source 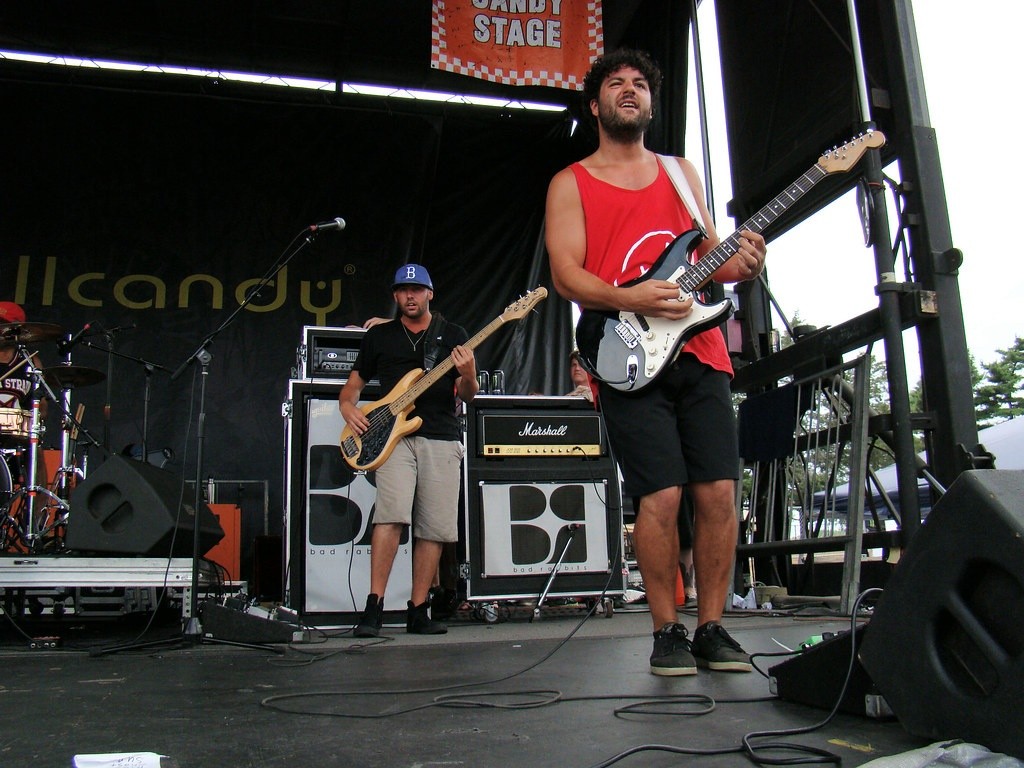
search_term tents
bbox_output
[812,415,1024,531]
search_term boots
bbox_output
[350,594,385,637]
[403,599,449,633]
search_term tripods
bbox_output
[90,232,321,661]
[0,335,87,557]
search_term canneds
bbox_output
[491,369,506,395]
[477,370,490,394]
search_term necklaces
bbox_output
[401,318,432,351]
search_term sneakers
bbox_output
[691,621,754,672]
[684,586,699,608]
[646,621,700,675]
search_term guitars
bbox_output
[572,125,891,395]
[339,282,550,473]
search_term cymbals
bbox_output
[40,364,109,389]
[0,322,65,349]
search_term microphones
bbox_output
[58,321,97,357]
[564,523,581,531]
[308,218,346,232]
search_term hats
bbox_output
[391,264,435,290]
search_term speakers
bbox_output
[280,376,432,628]
[450,395,628,601]
[64,452,225,560]
[856,469,1024,759]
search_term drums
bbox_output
[0,407,46,441]
[0,455,14,528]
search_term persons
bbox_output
[563,350,594,405]
[338,262,479,635]
[0,298,48,541]
[546,49,768,676]
